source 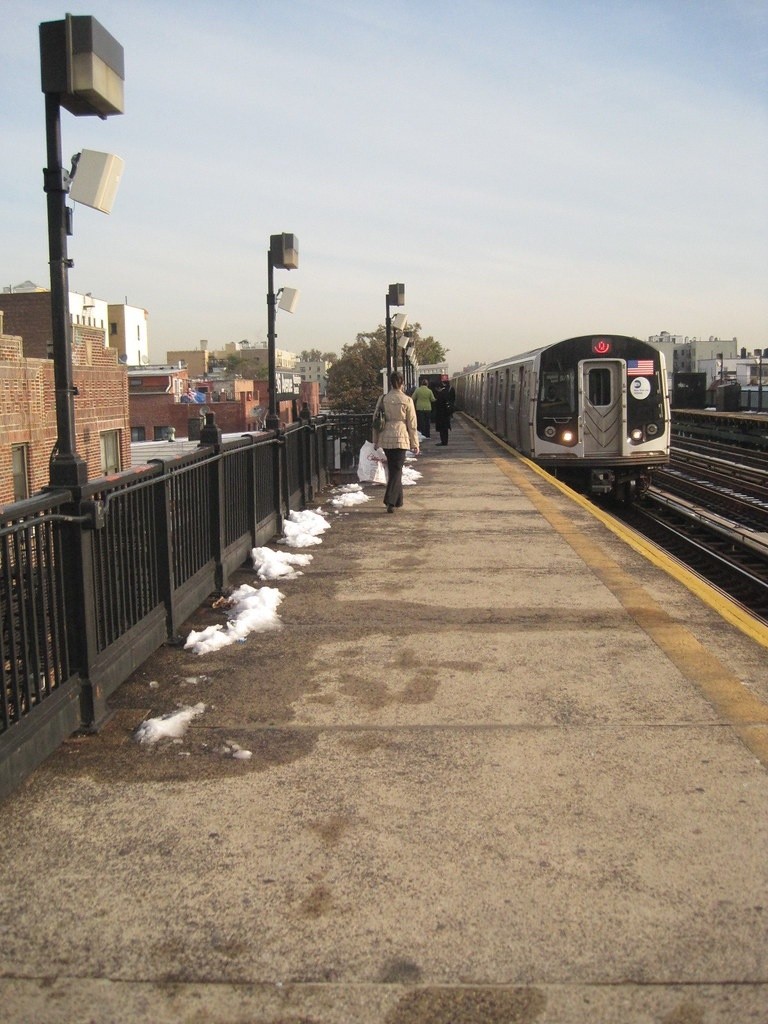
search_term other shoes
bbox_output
[435,442,445,446]
[387,502,393,513]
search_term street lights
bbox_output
[716,352,723,384]
[38,11,127,490]
[405,342,420,390]
[397,330,413,386]
[754,348,763,412]
[385,283,408,393]
[265,232,300,431]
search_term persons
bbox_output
[373,372,420,514]
[433,383,450,446]
[412,379,436,439]
[406,380,455,420]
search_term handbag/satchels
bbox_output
[358,440,388,487]
[372,394,386,433]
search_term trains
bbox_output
[449,334,673,504]
